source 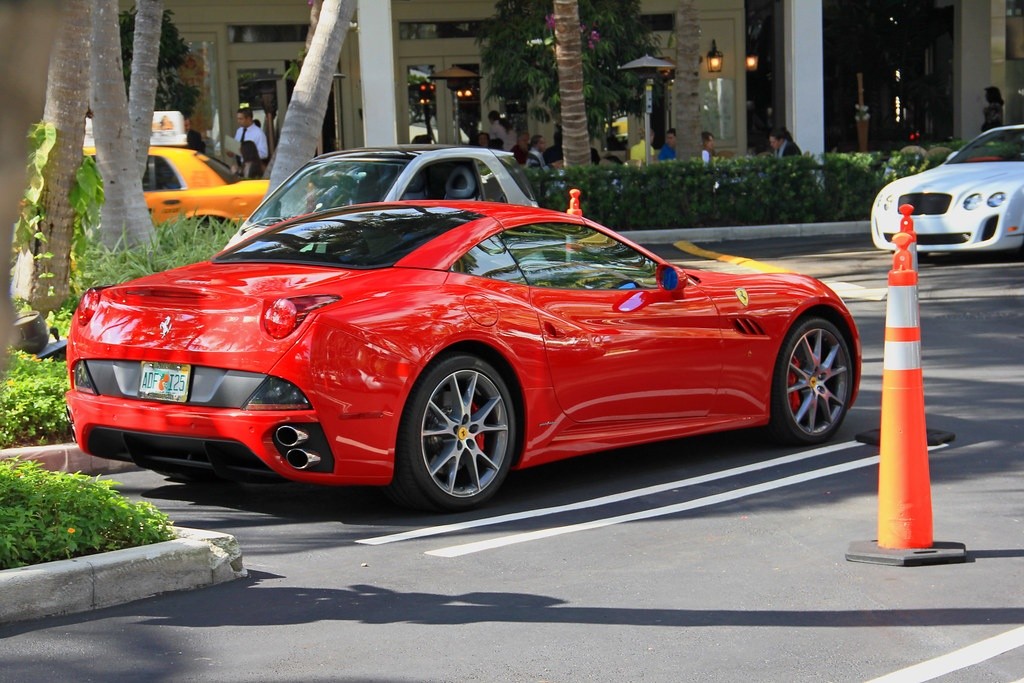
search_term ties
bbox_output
[240,127,247,143]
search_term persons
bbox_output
[183,117,207,155]
[701,132,716,165]
[224,107,269,179]
[629,127,655,164]
[981,86,1004,133]
[657,128,676,160]
[468,110,600,172]
[769,128,801,157]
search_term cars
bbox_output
[872,124,1024,266]
[84,111,269,234]
[222,145,540,253]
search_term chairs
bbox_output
[443,165,479,200]
[398,162,431,200]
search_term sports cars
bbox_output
[65,199,864,515]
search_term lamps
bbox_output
[427,64,483,91]
[707,39,723,73]
[745,48,759,69]
[241,73,285,93]
[619,53,676,79]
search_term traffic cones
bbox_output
[844,202,969,567]
[562,186,584,259]
